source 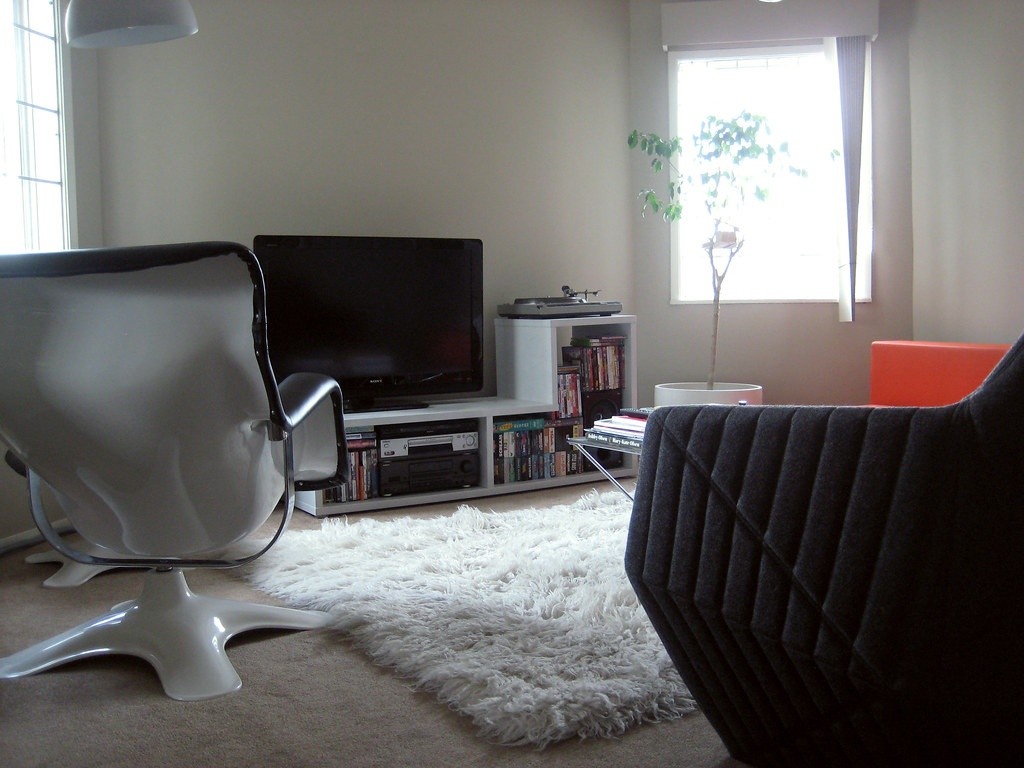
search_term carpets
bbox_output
[225,481,700,746]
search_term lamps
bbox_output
[64,0,199,47]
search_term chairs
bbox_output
[0,240,348,703]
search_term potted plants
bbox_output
[627,112,800,408]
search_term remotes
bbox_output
[621,407,652,418]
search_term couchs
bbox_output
[622,330,1024,768]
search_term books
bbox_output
[494,412,584,485]
[322,432,378,503]
[584,415,647,450]
[556,336,628,419]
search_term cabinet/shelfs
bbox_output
[294,313,638,519]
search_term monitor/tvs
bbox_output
[252,235,483,414]
